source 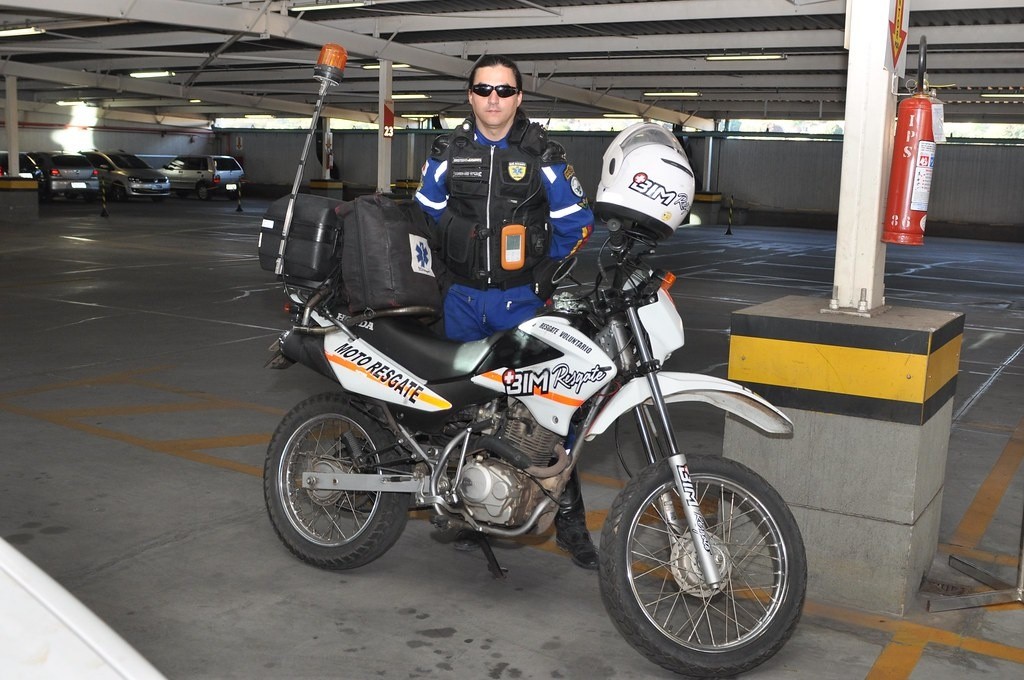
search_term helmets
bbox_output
[595,123,694,236]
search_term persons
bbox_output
[412,54,599,570]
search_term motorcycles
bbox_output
[255,43,810,680]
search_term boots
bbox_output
[545,457,602,570]
[454,520,489,551]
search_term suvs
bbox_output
[77,149,172,203]
[0,149,99,204]
[155,153,245,201]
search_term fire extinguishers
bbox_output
[881,36,945,246]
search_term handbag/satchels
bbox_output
[343,194,444,313]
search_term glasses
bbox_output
[471,83,518,98]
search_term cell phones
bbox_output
[501,225,525,270]
